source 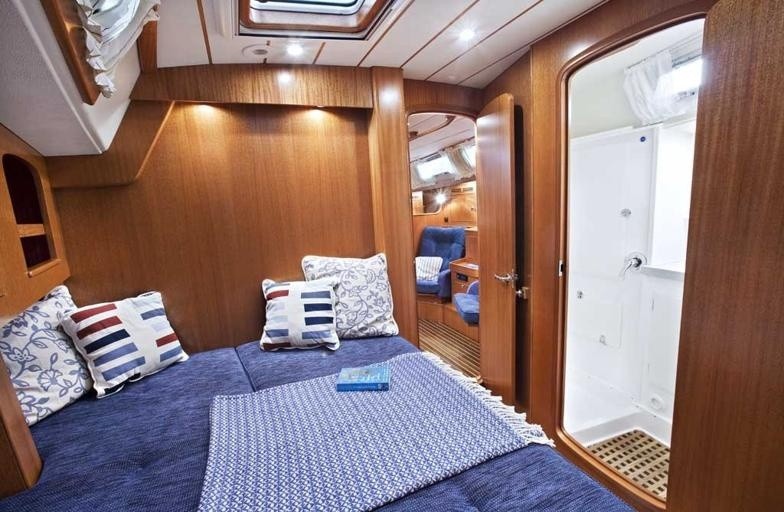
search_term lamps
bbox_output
[653,59,701,103]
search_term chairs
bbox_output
[454,281,483,324]
[416,226,465,299]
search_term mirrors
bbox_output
[642,120,699,279]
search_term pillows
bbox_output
[416,256,443,283]
[257,253,400,354]
[1,283,189,426]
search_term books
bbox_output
[336,365,390,391]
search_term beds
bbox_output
[0,318,637,510]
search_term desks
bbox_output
[449,257,481,306]
